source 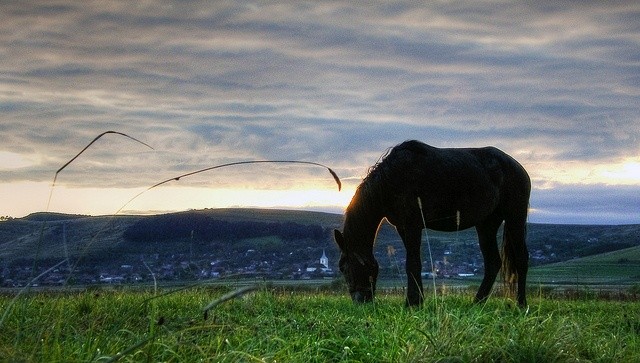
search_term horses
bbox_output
[334,139,531,309]
